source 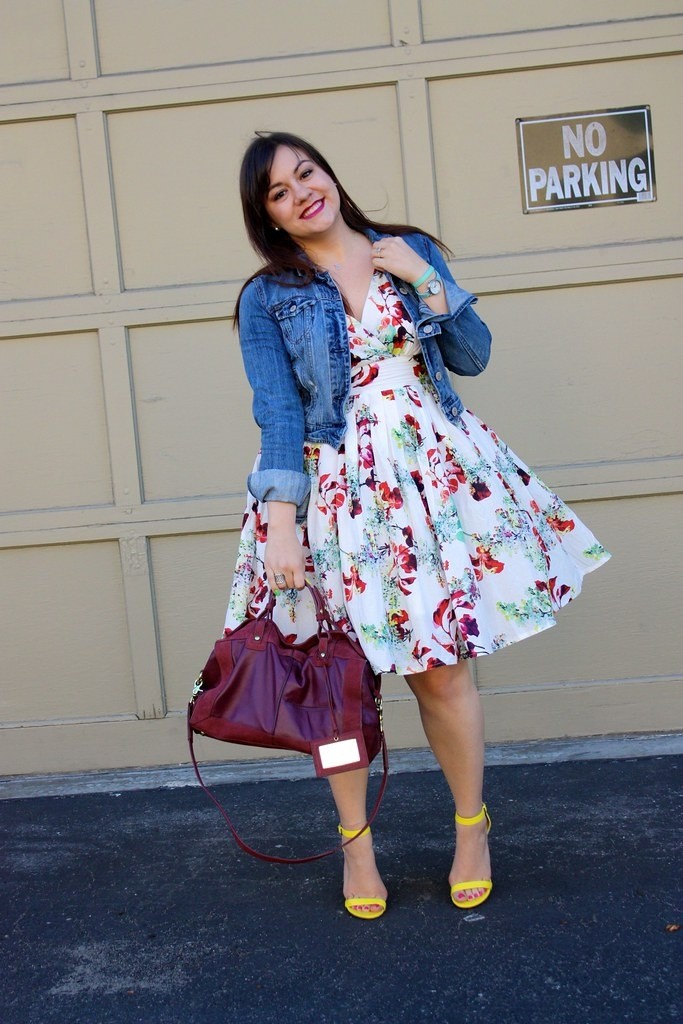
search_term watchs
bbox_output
[415,271,443,297]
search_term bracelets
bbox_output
[413,266,434,287]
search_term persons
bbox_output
[223,131,612,919]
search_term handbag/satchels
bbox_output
[186,579,388,865]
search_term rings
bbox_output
[275,574,283,583]
[375,247,382,257]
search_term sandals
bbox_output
[449,801,492,908]
[337,821,388,920]
